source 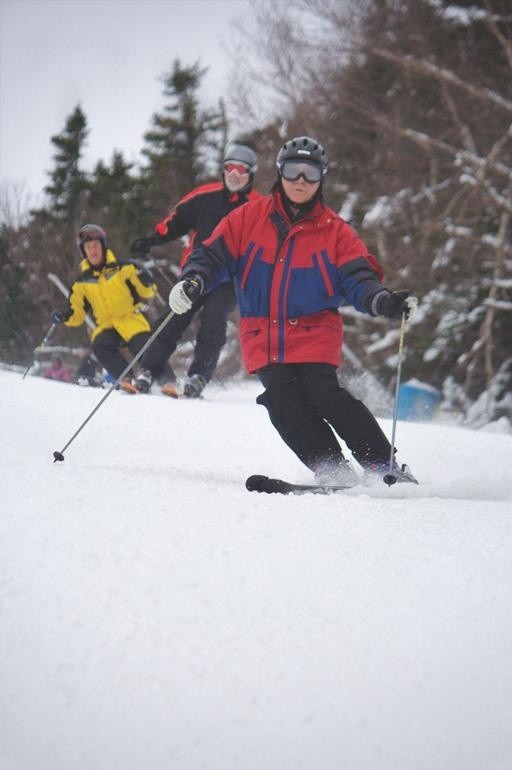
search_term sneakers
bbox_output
[113,368,210,397]
[315,455,361,485]
[362,457,402,485]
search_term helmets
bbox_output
[276,136,329,172]
[77,223,108,256]
[223,143,261,178]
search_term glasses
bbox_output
[276,158,326,183]
[225,159,258,174]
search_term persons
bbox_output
[130,143,266,397]
[73,348,98,387]
[51,223,185,398]
[44,356,69,383]
[169,136,423,488]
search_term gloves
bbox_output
[373,288,418,322]
[139,259,160,285]
[128,231,163,259]
[168,272,203,316]
[51,306,75,323]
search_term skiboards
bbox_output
[245,474,353,495]
[120,376,179,398]
